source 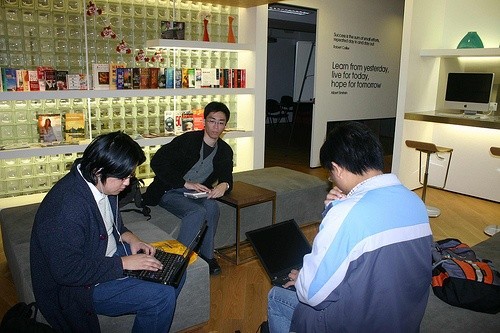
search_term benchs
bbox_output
[420,231,500,333]
[0,165,329,333]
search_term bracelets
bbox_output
[227,182,229,190]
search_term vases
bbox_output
[203,19,210,41]
[228,16,237,43]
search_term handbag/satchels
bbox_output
[431,238,500,314]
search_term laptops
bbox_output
[245,219,312,291]
[122,219,209,289]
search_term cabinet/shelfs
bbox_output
[0,38,257,206]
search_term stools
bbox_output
[482,146,500,236]
[214,180,277,266]
[406,139,454,216]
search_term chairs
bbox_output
[265,94,294,135]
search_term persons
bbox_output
[268,122,432,333]
[43,119,57,142]
[150,101,233,275]
[30,130,187,333]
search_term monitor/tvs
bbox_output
[444,72,495,112]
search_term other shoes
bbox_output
[199,254,220,272]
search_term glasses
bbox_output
[122,171,136,181]
[327,168,334,182]
[207,120,225,125]
